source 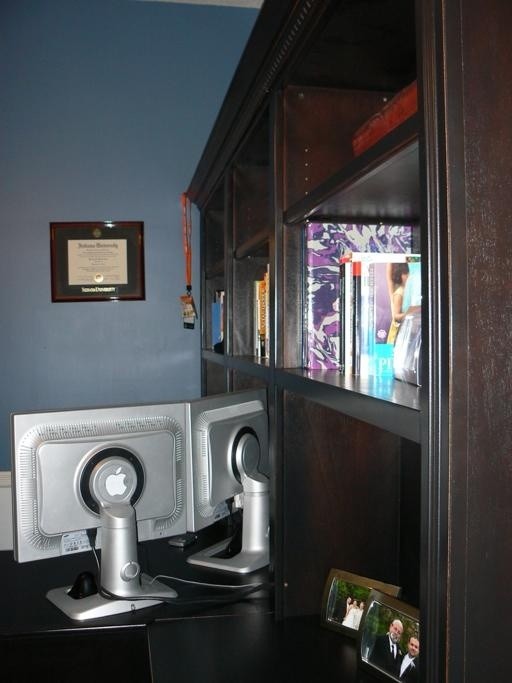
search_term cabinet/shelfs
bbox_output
[185,0,512,683]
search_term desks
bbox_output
[0,518,275,640]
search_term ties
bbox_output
[392,645,394,658]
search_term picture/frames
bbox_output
[319,568,402,641]
[49,221,145,303]
[357,589,420,683]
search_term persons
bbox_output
[367,618,406,679]
[390,635,418,683]
[340,599,360,628]
[331,595,353,623]
[351,601,367,630]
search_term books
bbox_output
[212,220,422,378]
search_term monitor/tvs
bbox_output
[185,385,271,575]
[9,399,188,621]
